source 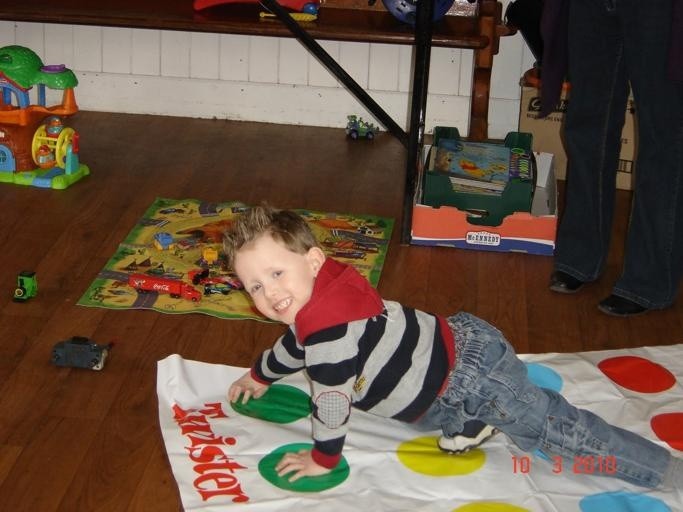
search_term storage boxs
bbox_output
[516,77,639,191]
[411,144,558,257]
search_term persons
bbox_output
[536,0,683,317]
[227,200,683,493]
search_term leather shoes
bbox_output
[597,295,648,317]
[550,271,584,294]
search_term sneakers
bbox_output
[437,420,501,455]
